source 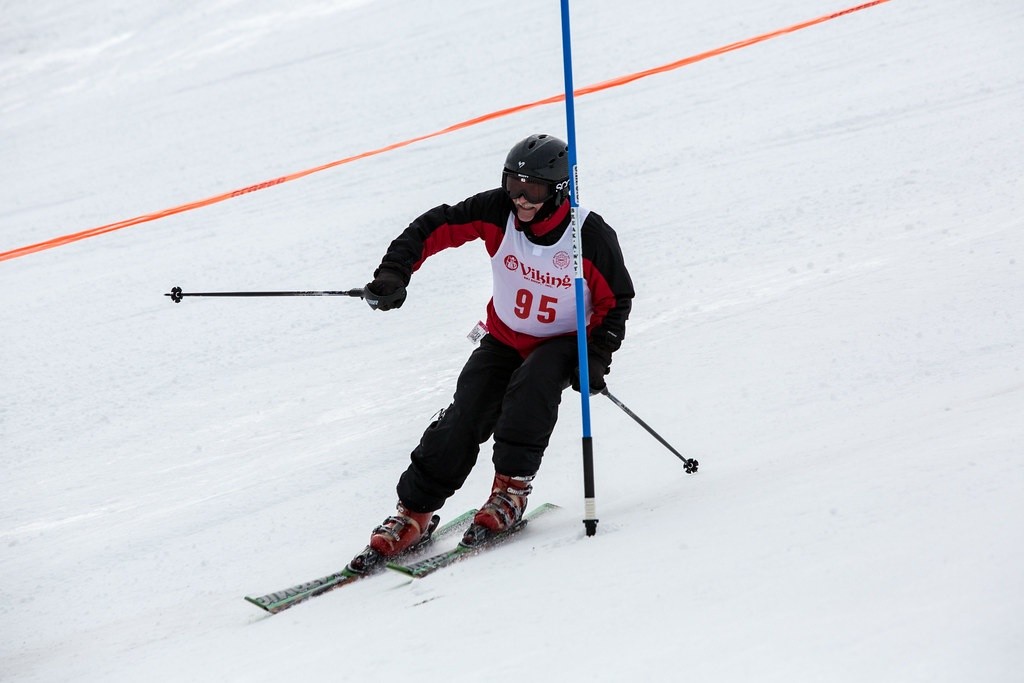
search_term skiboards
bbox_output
[244,502,567,617]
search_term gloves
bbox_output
[572,358,610,396]
[363,273,407,311]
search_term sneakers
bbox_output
[474,472,535,533]
[370,499,433,556]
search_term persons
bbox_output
[370,134,636,557]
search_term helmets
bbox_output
[504,134,570,195]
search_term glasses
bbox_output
[506,176,554,204]
